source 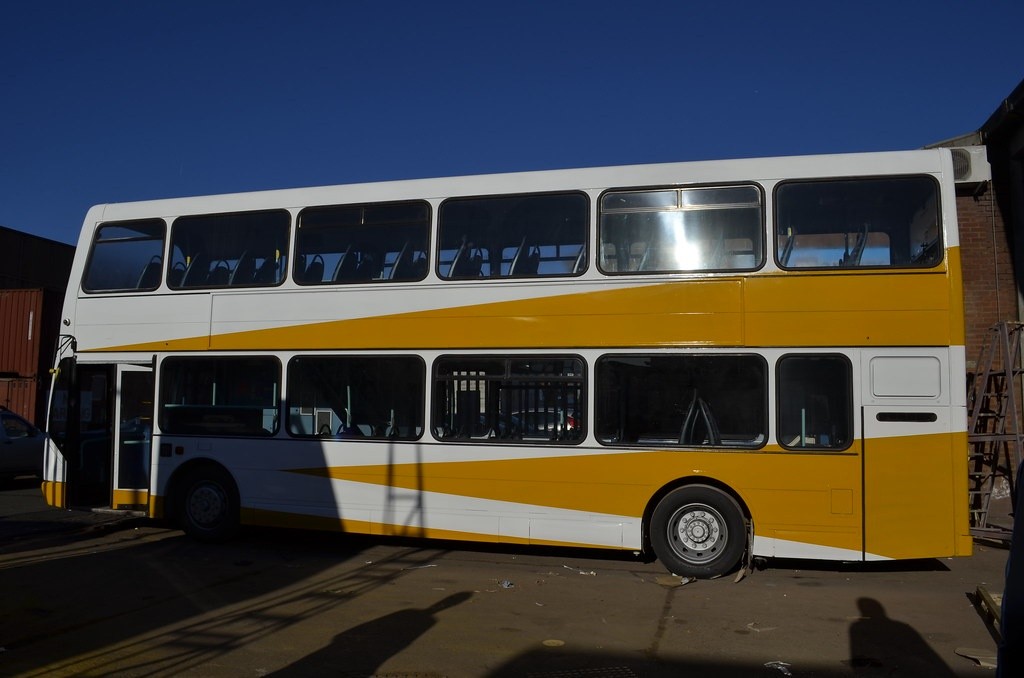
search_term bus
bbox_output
[41,150,972,581]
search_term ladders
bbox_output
[966,321,1024,542]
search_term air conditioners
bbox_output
[938,145,992,184]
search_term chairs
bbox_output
[129,215,871,443]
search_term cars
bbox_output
[1,405,43,478]
[511,410,581,432]
